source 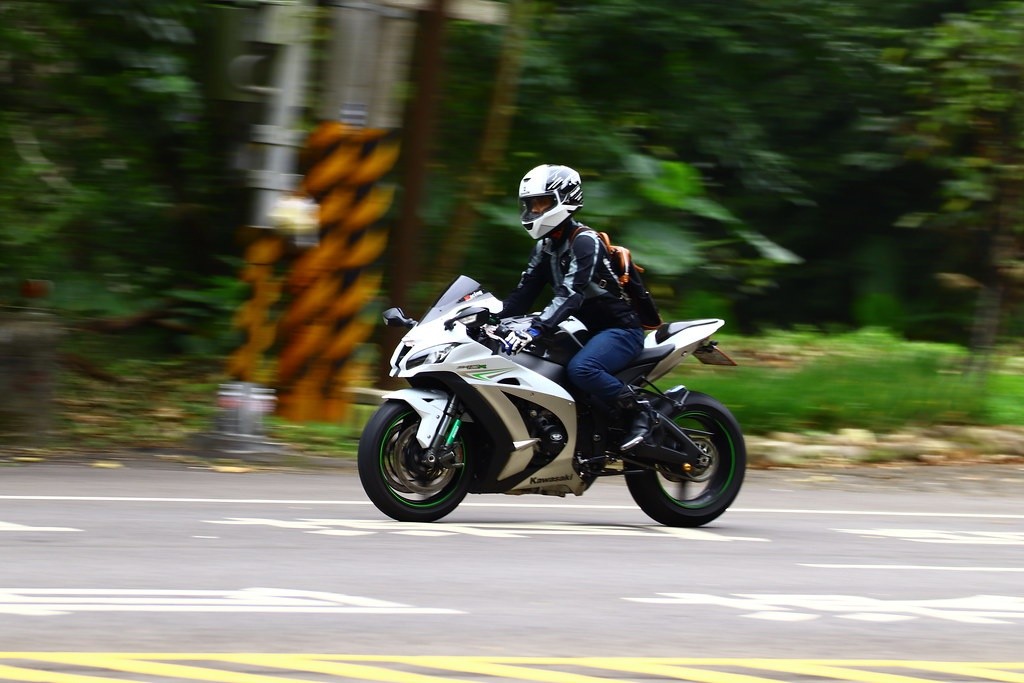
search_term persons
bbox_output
[499,164,660,453]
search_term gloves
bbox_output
[501,327,541,356]
[457,289,485,304]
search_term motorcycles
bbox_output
[357,275,746,528]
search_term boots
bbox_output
[610,384,662,451]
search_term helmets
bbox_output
[518,164,584,241]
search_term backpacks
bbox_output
[571,226,663,329]
